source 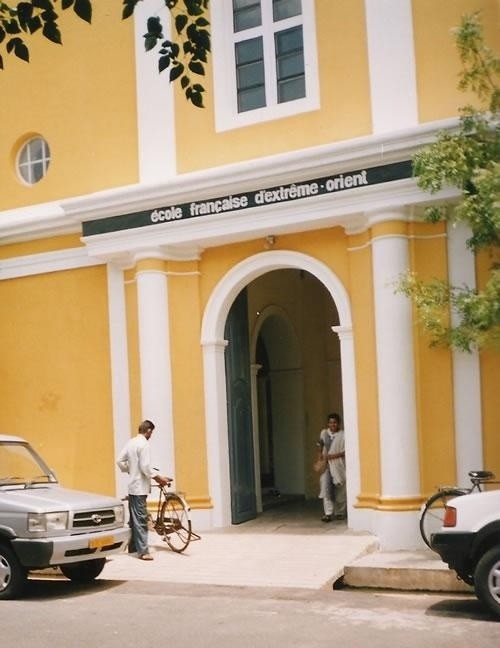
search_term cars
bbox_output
[429,489,500,618]
[0,435,126,599]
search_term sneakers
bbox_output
[321,515,345,521]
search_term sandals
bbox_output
[139,553,154,560]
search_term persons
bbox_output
[313,411,346,523]
[115,418,170,560]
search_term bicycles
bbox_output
[418,469,500,552]
[123,468,201,553]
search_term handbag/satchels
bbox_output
[313,458,327,473]
